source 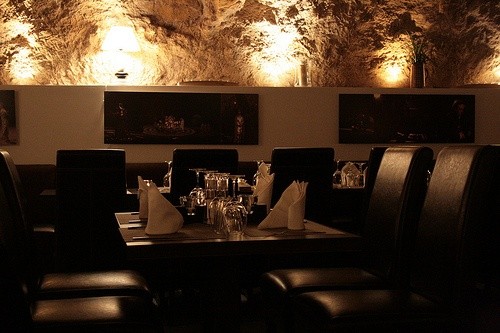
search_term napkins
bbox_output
[258,180,309,230]
[341,162,360,187]
[137,176,184,235]
[254,172,275,213]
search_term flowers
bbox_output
[387,12,445,64]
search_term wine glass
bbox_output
[189,168,248,237]
[164,160,171,189]
[355,162,366,188]
[333,158,342,187]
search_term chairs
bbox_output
[298,144,500,333]
[271,147,335,226]
[170,149,239,207]
[362,146,389,205]
[0,184,147,333]
[54,149,128,272]
[258,143,435,333]
[0,149,150,301]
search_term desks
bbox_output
[127,185,364,214]
[115,212,361,333]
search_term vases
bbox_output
[409,60,425,89]
